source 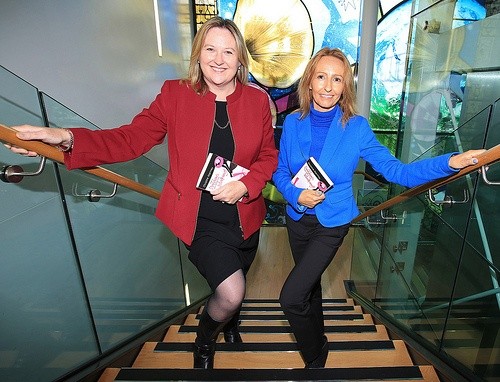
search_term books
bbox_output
[291,157,333,193]
[196,153,249,202]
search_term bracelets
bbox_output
[57,128,74,152]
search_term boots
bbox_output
[224,303,244,350]
[194,295,233,368]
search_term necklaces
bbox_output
[213,118,231,128]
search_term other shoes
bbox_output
[301,335,329,369]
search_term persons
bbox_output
[4,17,278,369]
[272,48,488,368]
